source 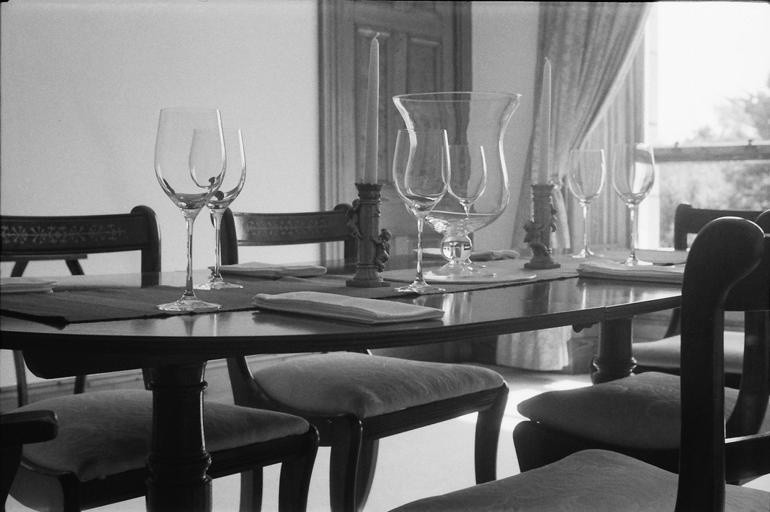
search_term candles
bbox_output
[364,31,380,184]
[538,57,551,185]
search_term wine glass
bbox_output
[566,143,656,266]
[392,91,523,295]
[154,105,247,312]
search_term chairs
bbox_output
[1,203,322,511]
[511,211,770,487]
[590,205,770,389]
[385,216,770,512]
[209,203,511,512]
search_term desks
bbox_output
[1,243,690,511]
